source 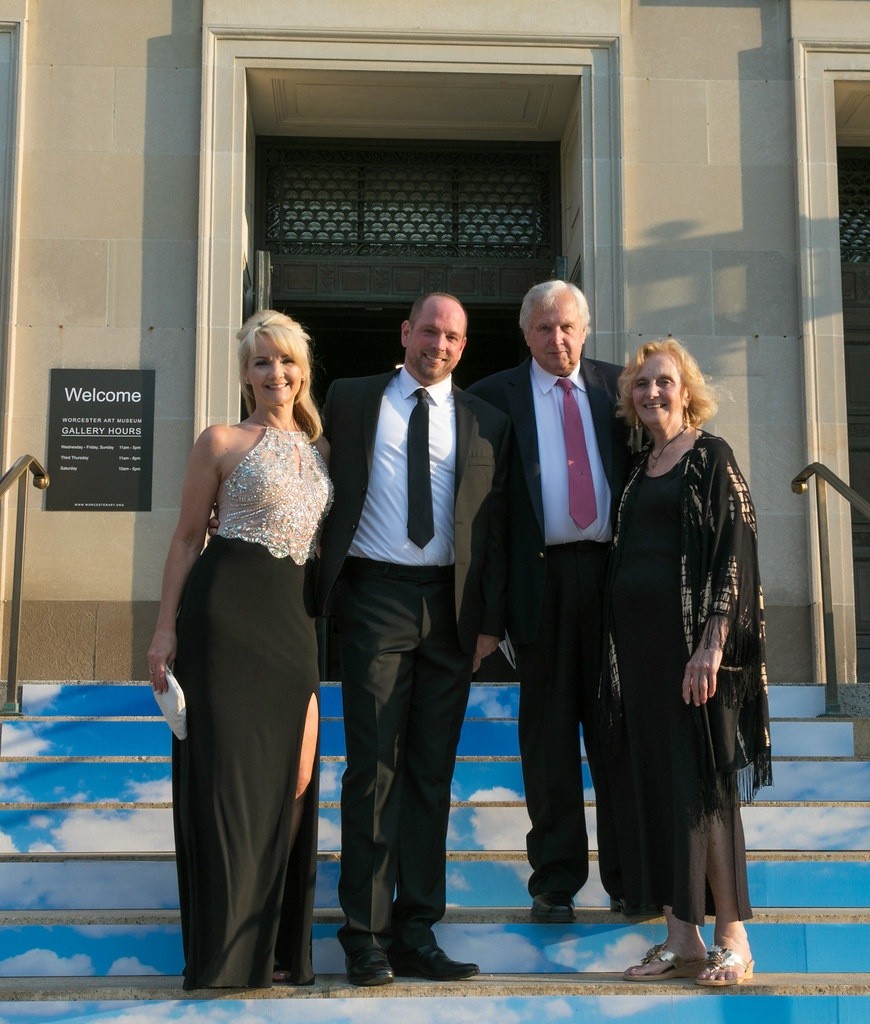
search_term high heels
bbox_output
[693,943,755,987]
[623,940,709,981]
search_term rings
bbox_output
[148,667,155,674]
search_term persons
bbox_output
[465,280,629,921]
[608,338,773,986]
[207,290,512,984]
[147,310,336,989]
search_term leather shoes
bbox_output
[384,938,480,981]
[531,891,576,923]
[610,895,665,917]
[345,949,395,987]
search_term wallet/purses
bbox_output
[152,664,188,740]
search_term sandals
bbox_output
[272,960,293,983]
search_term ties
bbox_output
[557,378,597,530]
[407,390,436,551]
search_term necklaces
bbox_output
[646,426,688,468]
